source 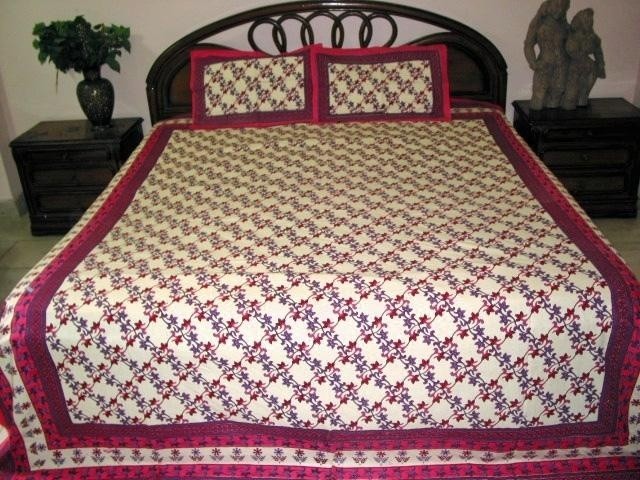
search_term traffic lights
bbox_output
[189,41,454,128]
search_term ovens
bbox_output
[0,1,640,479]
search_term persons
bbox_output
[560,7,605,110]
[523,0,573,110]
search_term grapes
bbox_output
[8,117,145,236]
[510,98,640,220]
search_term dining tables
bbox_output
[32,14,131,127]
